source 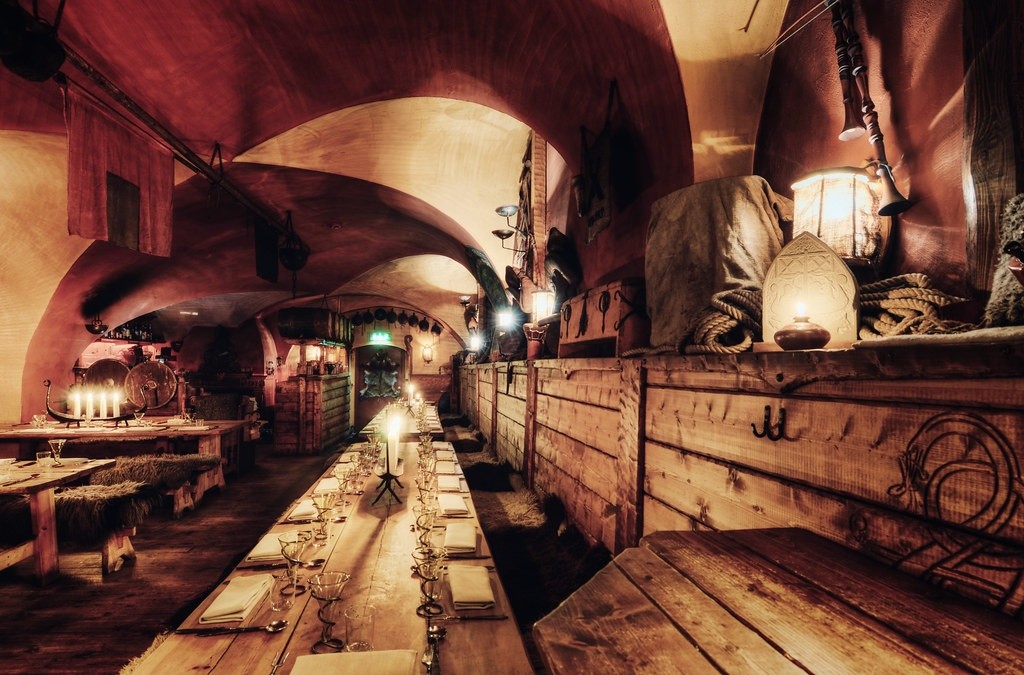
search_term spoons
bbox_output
[2,474,41,486]
[278,516,347,524]
[347,491,364,495]
[236,558,326,570]
[195,620,289,638]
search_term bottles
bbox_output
[297,360,344,375]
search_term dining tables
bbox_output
[0,418,250,519]
[359,404,444,442]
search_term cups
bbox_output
[36,452,51,469]
[196,419,204,427]
[270,570,297,612]
[344,605,377,653]
[144,420,152,427]
[334,491,346,520]
[33,415,46,430]
[95,421,104,430]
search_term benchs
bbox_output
[65,453,224,524]
[0,481,152,578]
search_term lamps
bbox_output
[495,206,526,238]
[492,229,526,253]
[773,302,831,351]
[458,295,471,308]
[422,345,433,364]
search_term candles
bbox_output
[86,393,93,419]
[74,394,80,419]
[100,394,106,419]
[384,416,401,472]
[113,393,120,417]
[408,384,414,406]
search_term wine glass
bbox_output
[134,413,145,427]
[277,427,382,593]
[306,571,350,654]
[82,415,93,428]
[48,439,66,465]
[412,398,447,604]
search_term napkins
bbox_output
[199,402,497,675]
[18,419,210,433]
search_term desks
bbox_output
[127,441,535,675]
[0,459,116,581]
[532,526,1024,675]
